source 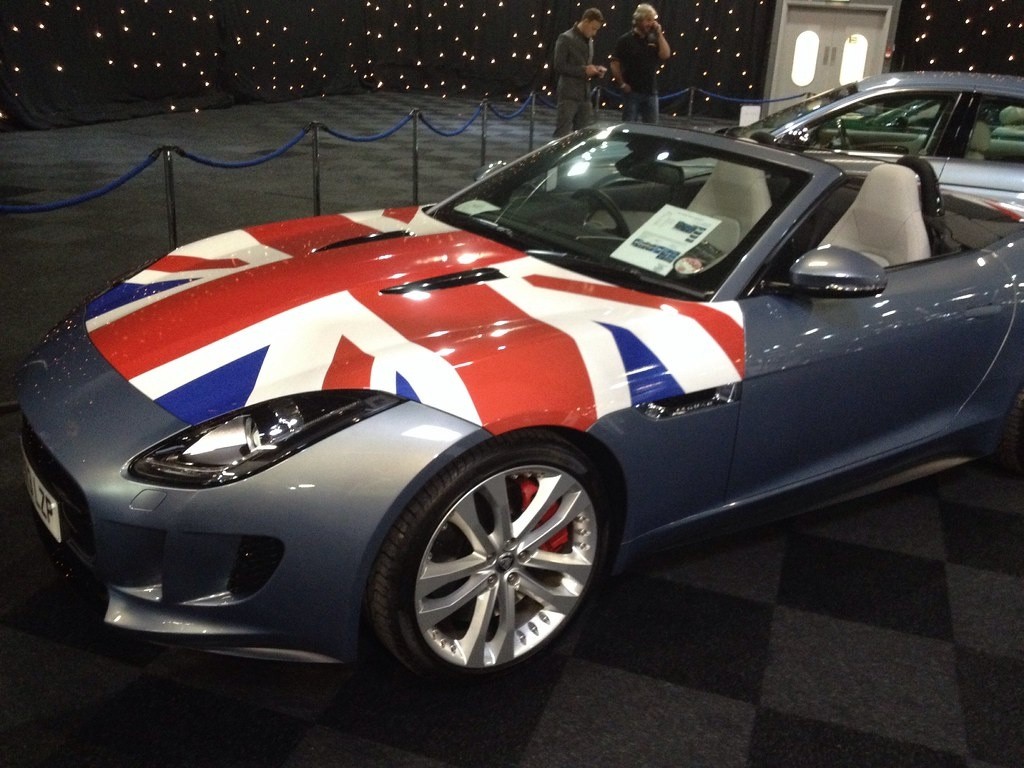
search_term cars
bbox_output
[15,122,1024,685]
[695,71,1024,217]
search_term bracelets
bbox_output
[620,83,626,89]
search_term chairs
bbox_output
[965,121,990,160]
[817,157,934,267]
[684,137,773,268]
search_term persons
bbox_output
[553,8,607,138]
[609,3,670,125]
